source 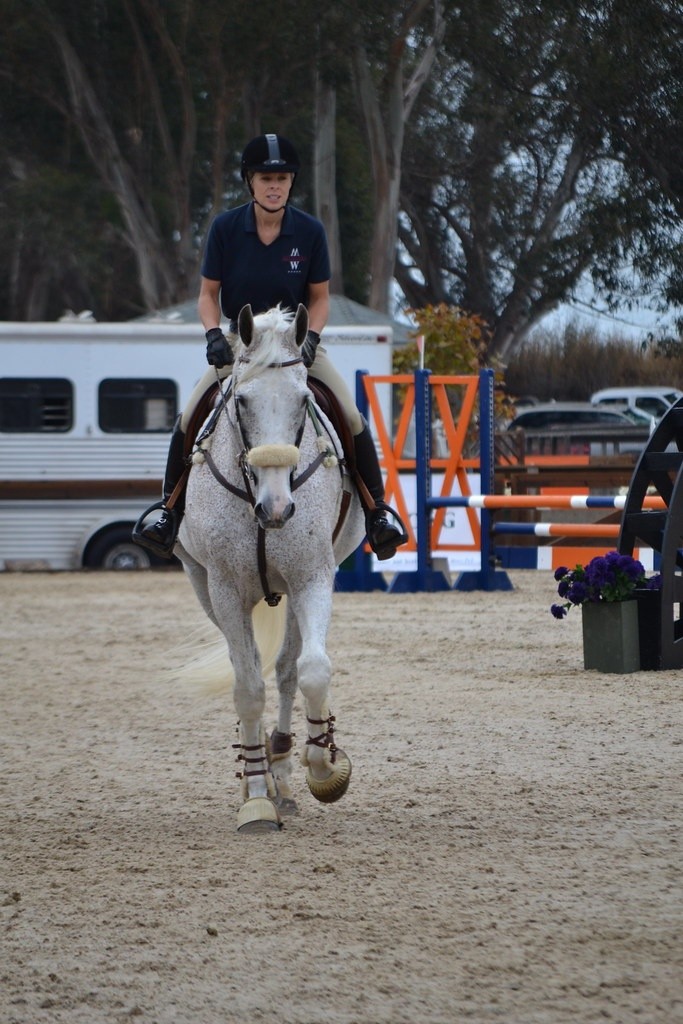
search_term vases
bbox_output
[582,601,641,675]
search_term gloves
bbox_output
[205,328,234,369]
[301,330,321,367]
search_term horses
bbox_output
[162,301,368,834]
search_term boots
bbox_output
[350,413,400,561]
[141,413,192,545]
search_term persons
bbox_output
[141,134,402,561]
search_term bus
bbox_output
[0,311,393,573]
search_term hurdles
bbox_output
[329,366,671,594]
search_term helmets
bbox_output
[240,134,299,182]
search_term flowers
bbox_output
[550,549,646,621]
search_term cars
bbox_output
[422,387,683,472]
